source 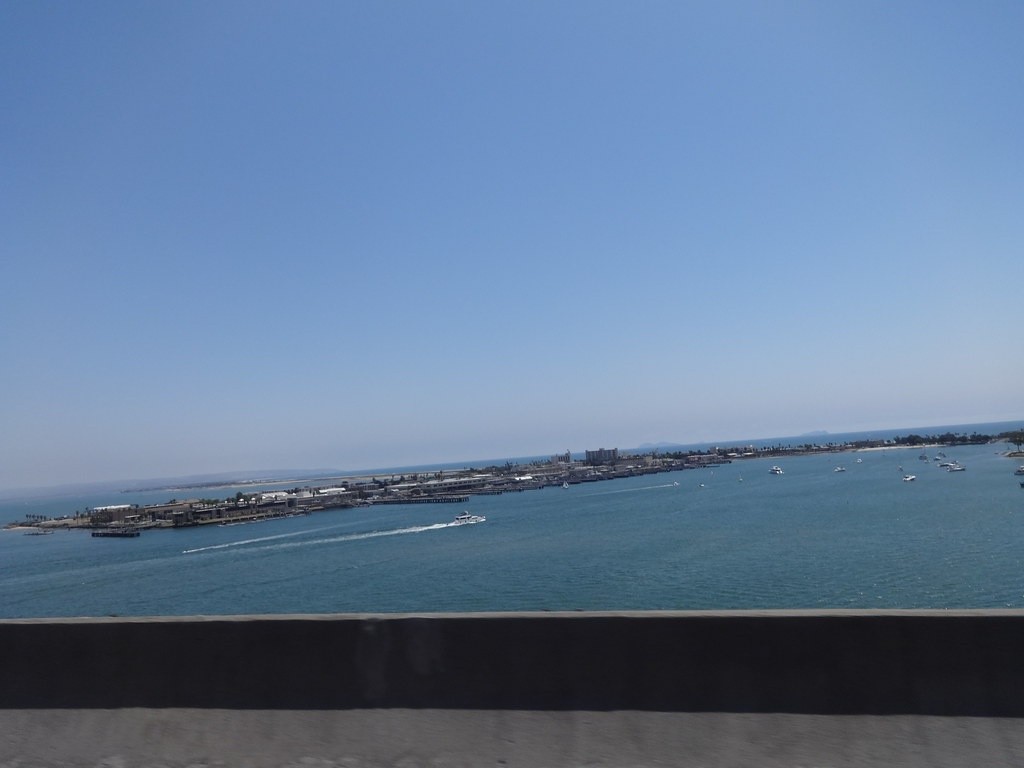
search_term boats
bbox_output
[673,482,678,486]
[833,466,846,472]
[700,483,704,487]
[903,474,916,481]
[919,450,966,472]
[768,464,785,474]
[454,510,486,524]
[854,458,862,463]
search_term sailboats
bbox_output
[739,471,743,481]
